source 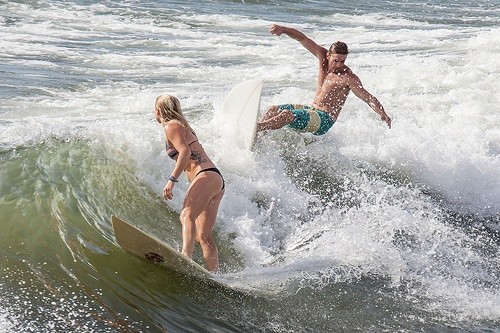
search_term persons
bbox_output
[156,93,226,274]
[257,24,393,136]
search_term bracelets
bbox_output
[170,176,180,183]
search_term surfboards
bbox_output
[209,78,262,151]
[109,213,214,280]
